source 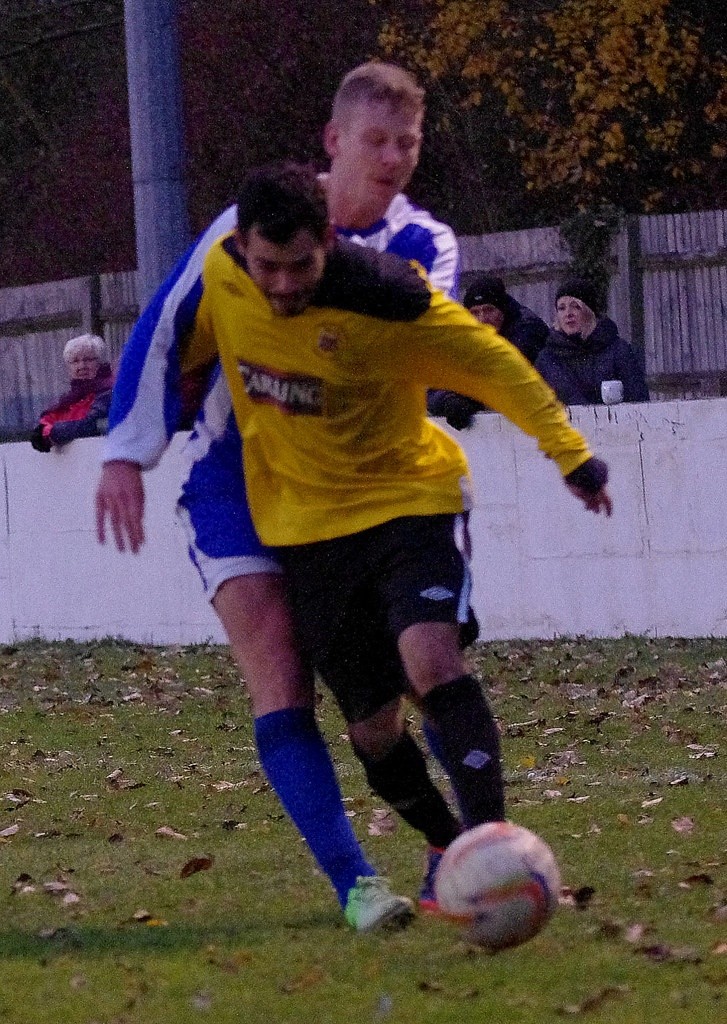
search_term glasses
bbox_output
[67,356,98,367]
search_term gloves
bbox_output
[30,424,52,454]
[445,401,485,430]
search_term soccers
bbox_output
[435,821,560,949]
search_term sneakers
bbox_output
[343,876,414,934]
[417,843,448,911]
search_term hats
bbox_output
[464,277,508,316]
[555,280,601,320]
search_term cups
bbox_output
[601,380,623,405]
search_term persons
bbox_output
[30,335,114,452]
[96,64,461,933]
[536,280,649,406]
[427,277,550,430]
[189,164,612,910]
